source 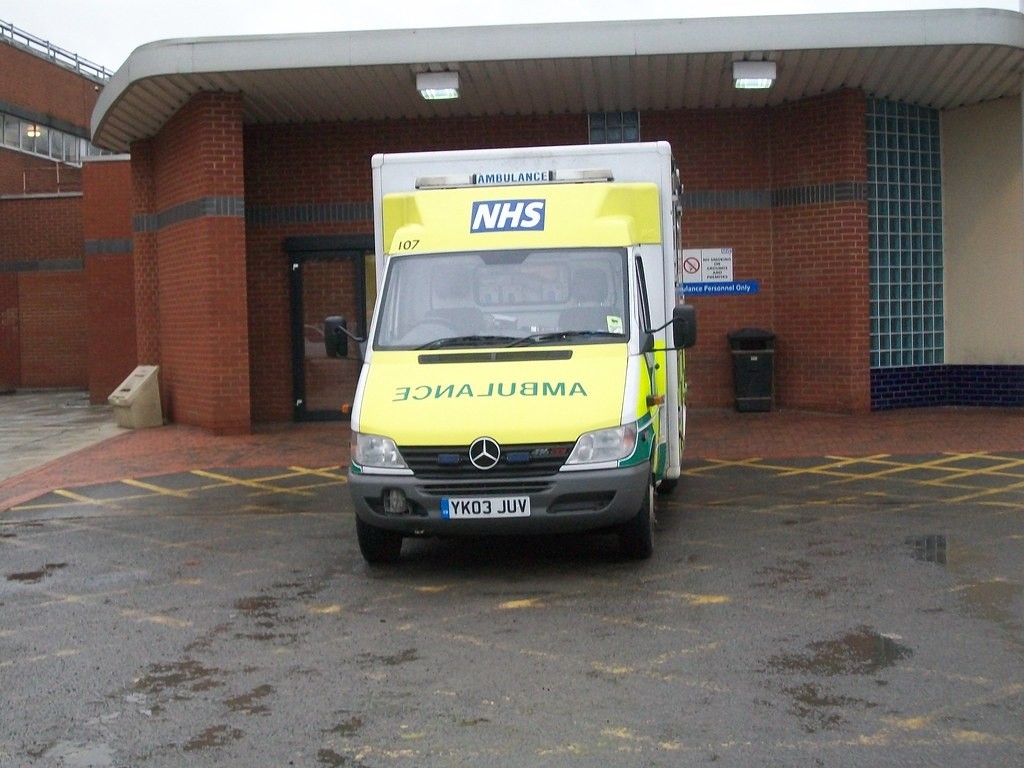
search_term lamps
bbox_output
[25,124,41,137]
[414,71,463,102]
[732,60,776,91]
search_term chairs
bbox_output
[558,269,620,333]
[425,280,484,336]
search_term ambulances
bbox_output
[323,141,696,575]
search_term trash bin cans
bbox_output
[724,328,778,415]
